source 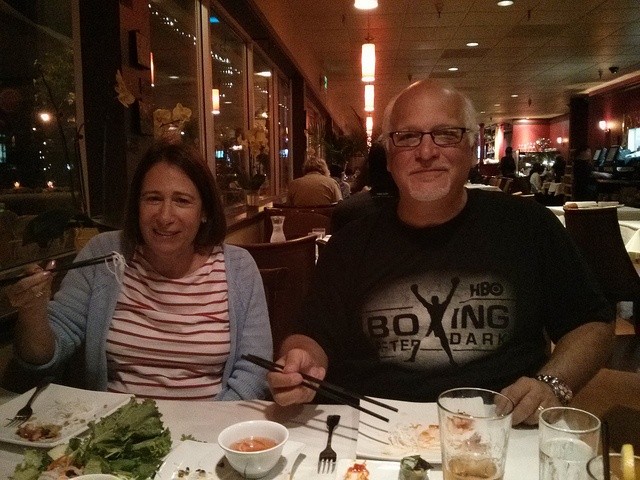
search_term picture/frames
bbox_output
[604,144,621,165]
[592,147,604,163]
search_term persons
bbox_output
[255,145,270,177]
[551,155,566,183]
[267,77,616,428]
[285,157,343,208]
[4,138,275,402]
[572,146,601,201]
[329,134,393,235]
[500,145,516,178]
[529,162,542,194]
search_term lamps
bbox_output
[366,118,373,137]
[212,89,220,114]
[557,137,562,144]
[599,121,606,131]
[360,43,377,84]
[364,84,375,113]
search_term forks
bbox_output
[12,378,50,422]
[320,415,341,463]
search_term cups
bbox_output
[437,387,514,480]
[586,453,640,480]
[539,407,602,480]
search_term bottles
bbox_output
[270,216,287,244]
[398,456,428,480]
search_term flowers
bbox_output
[224,122,271,189]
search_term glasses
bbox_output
[389,127,470,147]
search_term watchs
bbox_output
[532,372,574,405]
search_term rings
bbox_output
[538,406,544,413]
[36,290,44,299]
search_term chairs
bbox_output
[226,234,318,328]
[263,207,334,237]
[527,180,565,202]
[314,240,325,255]
[561,206,639,302]
[478,175,514,191]
[252,267,290,346]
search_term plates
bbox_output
[356,396,497,464]
[0,383,135,448]
[153,440,306,480]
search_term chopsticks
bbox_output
[241,354,398,423]
[0,253,117,288]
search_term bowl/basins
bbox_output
[218,420,289,479]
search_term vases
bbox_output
[245,187,261,208]
[268,214,287,243]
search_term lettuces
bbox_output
[49,397,173,480]
